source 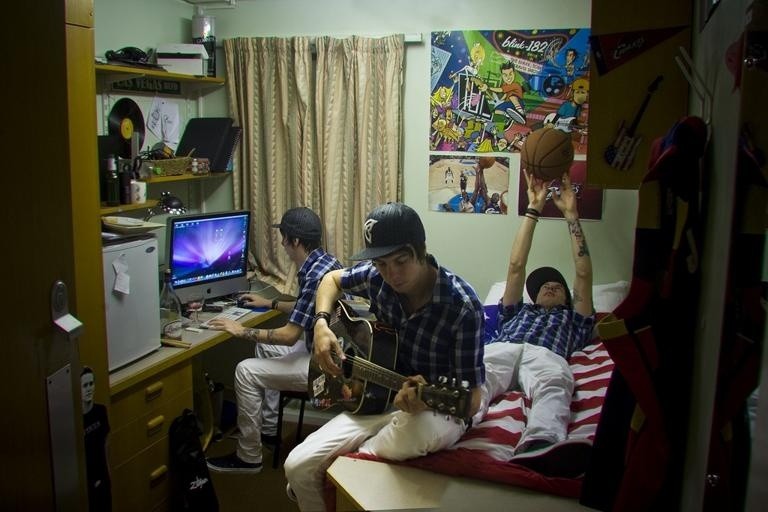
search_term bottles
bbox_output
[159,269,182,342]
[105,155,119,207]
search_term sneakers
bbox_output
[262,434,276,447]
[509,439,593,479]
[205,453,263,473]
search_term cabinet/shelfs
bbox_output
[91,62,233,215]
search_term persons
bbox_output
[206,207,355,472]
[284,202,485,512]
[458,160,481,213]
[460,170,467,199]
[473,167,597,479]
[549,46,579,85]
[479,167,501,214]
[544,78,590,140]
[443,203,454,212]
[431,47,526,152]
[79,367,112,511]
[445,168,454,187]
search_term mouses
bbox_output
[237,299,251,308]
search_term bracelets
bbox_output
[314,315,332,330]
[270,299,279,310]
[522,215,540,222]
[524,208,539,214]
[314,311,332,317]
[276,300,279,312]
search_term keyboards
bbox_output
[199,306,252,330]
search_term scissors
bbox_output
[123,157,141,181]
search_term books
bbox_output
[176,118,243,172]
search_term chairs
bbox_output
[273,390,310,470]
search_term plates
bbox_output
[106,216,144,225]
[103,216,167,235]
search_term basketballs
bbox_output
[521,128,573,179]
[480,156,495,168]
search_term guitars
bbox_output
[308,299,471,425]
[604,76,663,170]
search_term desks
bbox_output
[107,295,285,511]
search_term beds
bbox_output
[326,282,637,510]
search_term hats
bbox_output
[271,207,322,241]
[526,267,572,303]
[349,203,425,262]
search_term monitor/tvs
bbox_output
[166,210,251,313]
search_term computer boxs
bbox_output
[101,234,161,374]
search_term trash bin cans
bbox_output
[210,383,225,426]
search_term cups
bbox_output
[130,181,147,204]
[118,171,129,205]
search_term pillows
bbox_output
[479,279,630,317]
